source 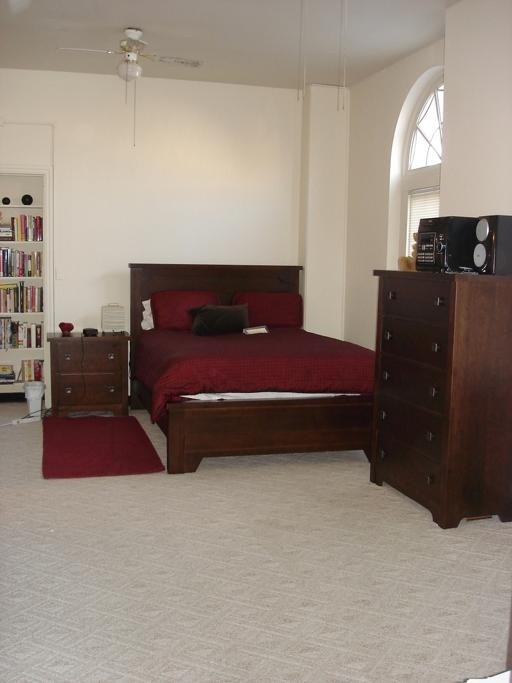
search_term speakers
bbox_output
[473,214,512,275]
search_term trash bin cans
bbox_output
[23,381,46,416]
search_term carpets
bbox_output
[40,417,167,480]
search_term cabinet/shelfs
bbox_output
[0,120,52,408]
[366,266,512,533]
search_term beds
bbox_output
[127,261,378,476]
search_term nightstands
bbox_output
[45,330,134,418]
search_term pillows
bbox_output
[140,287,306,334]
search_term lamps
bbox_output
[95,267,127,336]
[118,62,143,82]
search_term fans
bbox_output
[61,26,199,67]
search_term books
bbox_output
[0,215,43,384]
